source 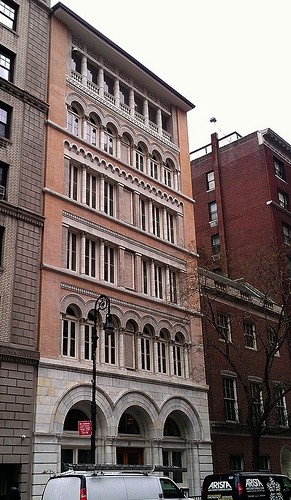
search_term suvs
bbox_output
[39,464,194,500]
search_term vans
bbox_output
[201,472,291,500]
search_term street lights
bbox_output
[88,293,113,463]
